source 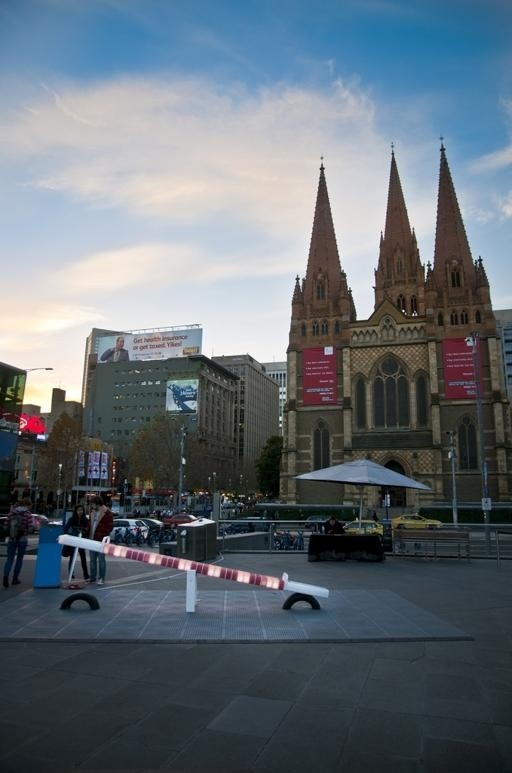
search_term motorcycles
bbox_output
[269,528,307,550]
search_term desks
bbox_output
[308,533,386,562]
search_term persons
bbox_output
[83,496,113,586]
[100,336,129,362]
[3,498,33,589]
[323,517,346,535]
[64,504,90,580]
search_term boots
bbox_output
[11,575,22,585]
[3,575,10,587]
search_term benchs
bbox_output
[392,527,471,564]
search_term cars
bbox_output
[392,513,443,530]
[110,513,249,535]
[28,514,50,533]
[343,517,384,543]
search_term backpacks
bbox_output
[7,509,29,541]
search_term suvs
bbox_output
[304,514,331,528]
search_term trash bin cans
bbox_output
[176,518,217,562]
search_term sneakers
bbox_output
[82,577,105,586]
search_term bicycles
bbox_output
[114,524,178,547]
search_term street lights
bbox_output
[56,462,63,518]
[465,329,493,557]
[446,429,459,528]
[178,424,190,513]
[207,475,211,493]
[239,471,247,491]
[9,362,57,433]
[212,470,217,493]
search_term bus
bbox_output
[98,492,243,519]
[252,503,361,526]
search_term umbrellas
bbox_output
[291,459,435,535]
